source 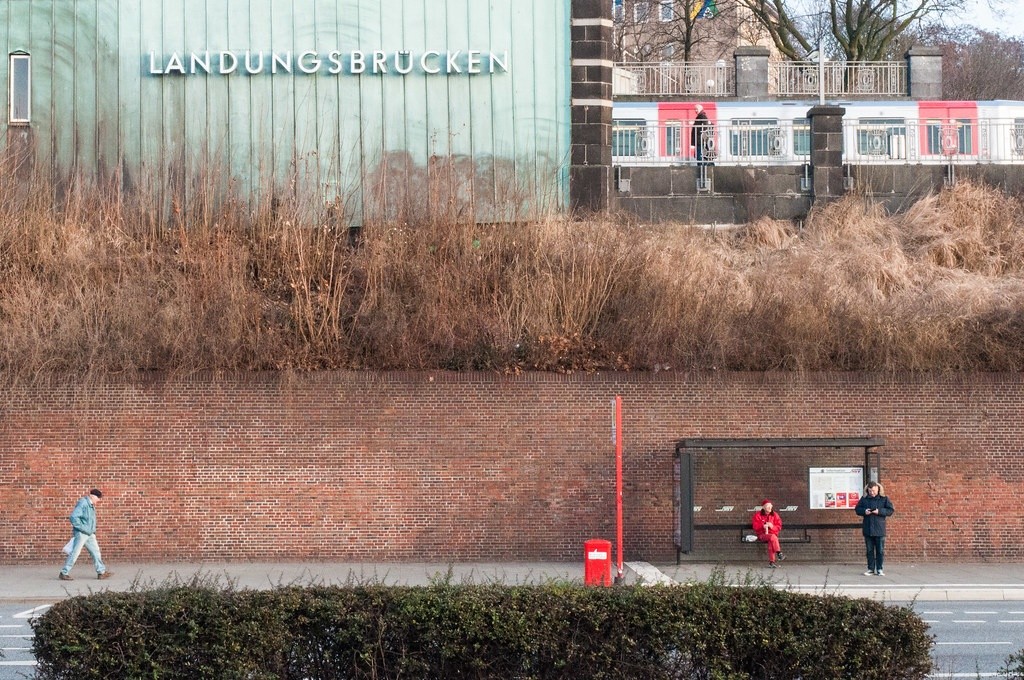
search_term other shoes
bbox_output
[777,554,787,562]
[768,561,777,568]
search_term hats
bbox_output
[762,499,772,506]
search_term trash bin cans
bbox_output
[583,539,613,588]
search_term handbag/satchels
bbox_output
[61,537,77,555]
[746,535,757,542]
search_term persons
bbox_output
[59,489,113,580]
[691,104,715,166]
[855,481,894,576]
[752,500,786,568]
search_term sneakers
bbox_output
[98,570,112,580]
[877,569,886,576]
[863,570,874,576]
[59,572,74,581]
